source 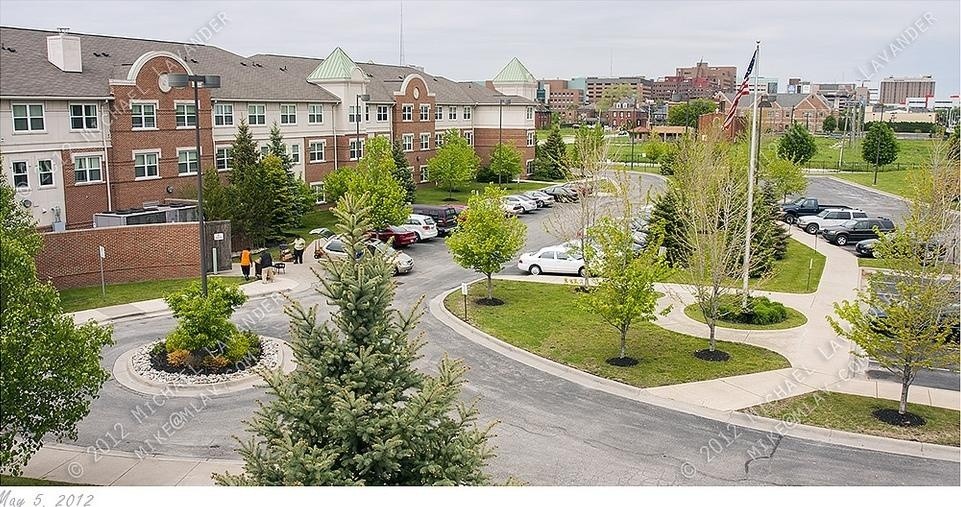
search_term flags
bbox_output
[721,47,757,133]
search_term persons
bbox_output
[292,235,306,264]
[260,250,276,283]
[239,247,254,281]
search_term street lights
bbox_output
[356,95,371,162]
[756,94,777,185]
[499,98,511,189]
[673,94,690,135]
[167,72,220,299]
[875,102,884,183]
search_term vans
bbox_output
[403,204,458,243]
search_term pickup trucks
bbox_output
[857,232,946,259]
[777,197,853,225]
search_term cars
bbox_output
[518,216,649,277]
[500,182,595,218]
[309,227,418,276]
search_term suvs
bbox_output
[796,208,867,235]
[824,218,895,245]
